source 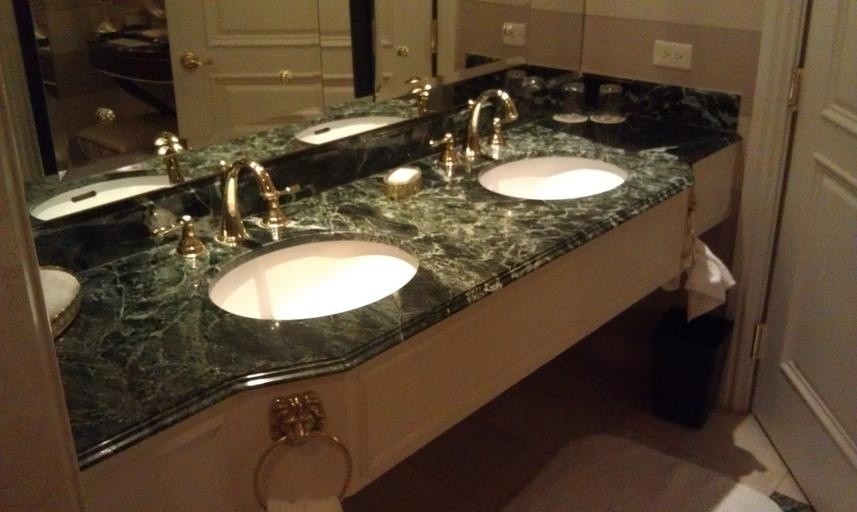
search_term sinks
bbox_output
[478,151,630,201]
[29,170,193,222]
[208,231,422,321]
[293,111,415,145]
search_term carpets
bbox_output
[503,424,785,512]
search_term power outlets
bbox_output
[652,39,691,69]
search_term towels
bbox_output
[682,227,738,326]
[259,497,343,512]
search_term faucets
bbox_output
[462,89,519,157]
[408,81,431,116]
[217,159,290,244]
[156,131,184,185]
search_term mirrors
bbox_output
[2,1,585,227]
